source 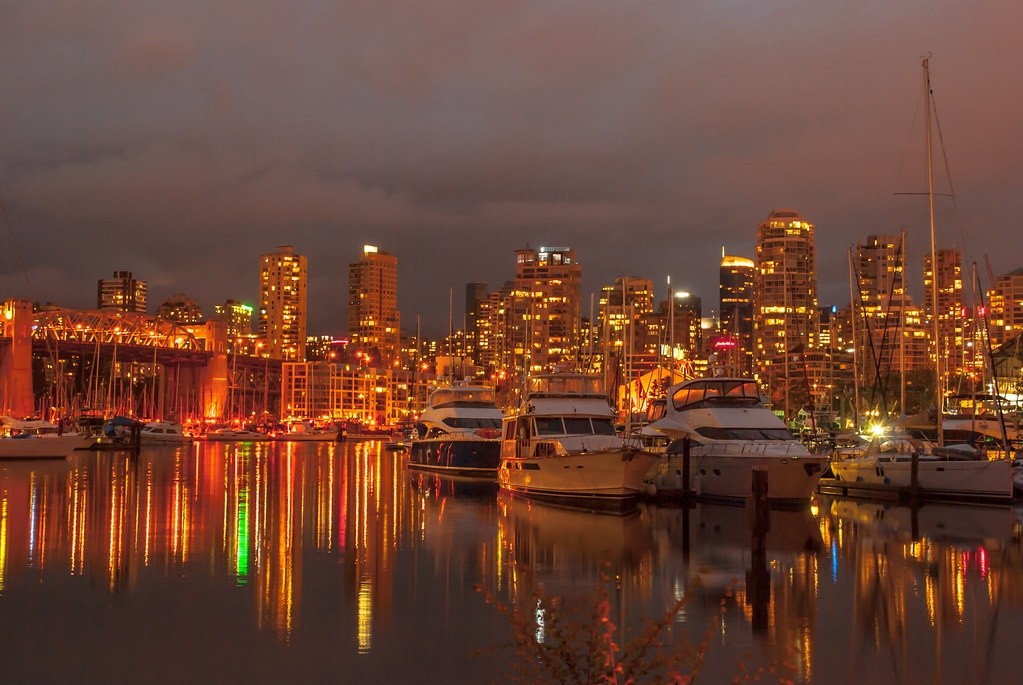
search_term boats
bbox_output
[134,343,193,441]
[206,429,274,443]
[399,287,504,486]
[502,278,659,518]
[67,338,146,451]
[275,427,339,441]
[0,419,80,460]
[618,272,836,511]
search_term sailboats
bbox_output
[791,54,1016,510]
[925,260,1023,497]
